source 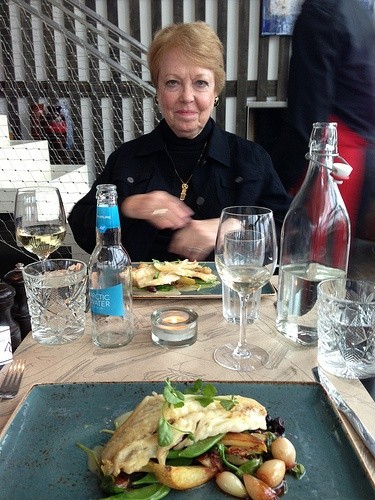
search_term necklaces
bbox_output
[173,141,207,200]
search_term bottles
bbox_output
[87,183,134,349]
[276,120,352,346]
[41,105,67,149]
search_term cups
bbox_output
[22,258,87,347]
[150,306,198,348]
[222,231,263,327]
[316,278,375,381]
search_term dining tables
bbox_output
[0,299,375,483]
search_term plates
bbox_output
[0,382,375,500]
[131,262,276,299]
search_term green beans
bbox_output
[100,433,261,500]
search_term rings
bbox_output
[193,246,202,253]
[152,208,168,217]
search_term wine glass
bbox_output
[213,205,278,372]
[14,186,67,273]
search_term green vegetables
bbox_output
[152,259,213,291]
[76,376,306,480]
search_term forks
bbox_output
[0,360,25,403]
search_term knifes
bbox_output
[313,367,375,461]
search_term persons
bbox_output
[287,0,375,272]
[67,20,311,275]
